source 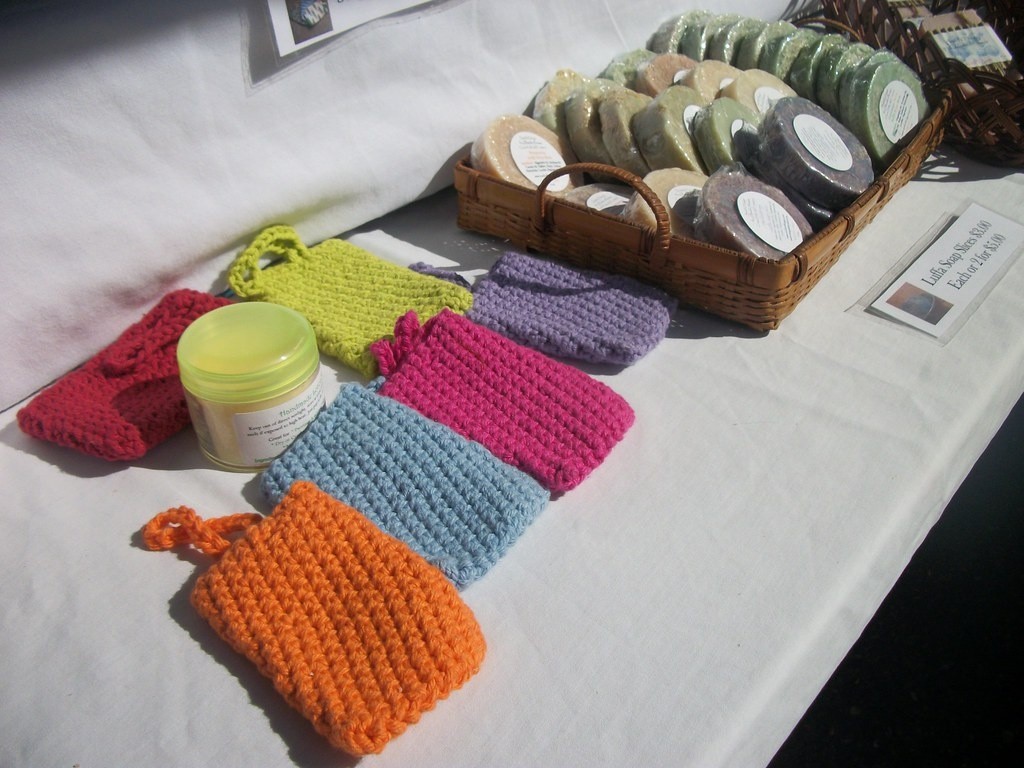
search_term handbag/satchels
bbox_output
[258,376,551,592]
[141,480,487,758]
[370,308,635,501]
[17,288,239,463]
[409,251,679,368]
[227,225,473,378]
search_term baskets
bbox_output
[821,0,1024,169]
[453,17,952,334]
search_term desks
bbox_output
[0,148,1024,768]
